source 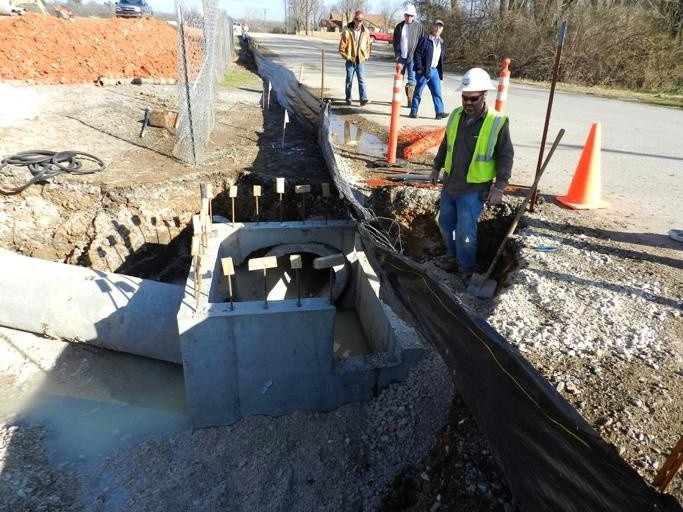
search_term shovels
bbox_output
[466,128,566,299]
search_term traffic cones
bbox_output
[493,58,511,112]
[372,64,409,168]
[556,121,612,210]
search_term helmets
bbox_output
[404,3,417,16]
[431,19,444,27]
[455,68,496,92]
[354,11,364,21]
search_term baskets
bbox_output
[148,109,179,128]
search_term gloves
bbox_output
[429,172,439,187]
[487,187,502,206]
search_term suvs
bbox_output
[115,0,153,19]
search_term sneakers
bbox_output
[461,270,474,287]
[437,256,456,269]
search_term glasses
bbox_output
[462,93,484,102]
[406,15,413,17]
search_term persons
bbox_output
[243,24,249,40]
[338,11,371,107]
[429,68,514,290]
[409,20,449,120]
[393,4,424,107]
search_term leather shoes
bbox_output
[436,113,450,120]
[410,112,418,118]
[361,100,368,106]
[346,100,350,104]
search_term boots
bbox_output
[405,83,416,107]
